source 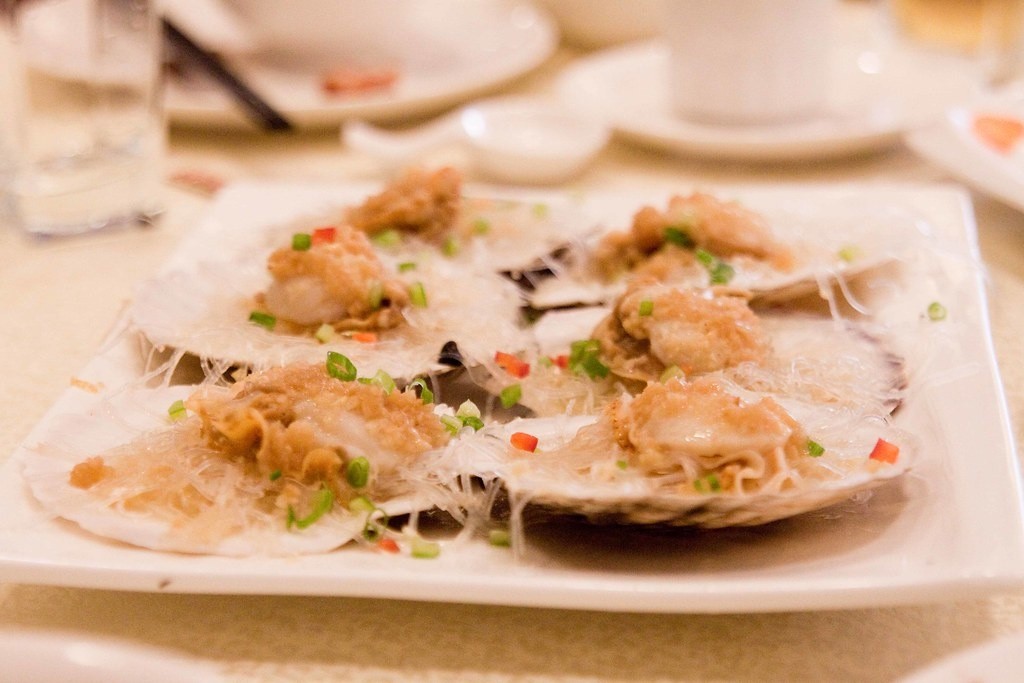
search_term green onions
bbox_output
[243,216,822,560]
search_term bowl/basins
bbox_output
[461,102,608,184]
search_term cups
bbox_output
[2,2,171,234]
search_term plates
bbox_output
[908,83,1024,209]
[553,41,982,162]
[165,2,561,131]
[1,172,1022,614]
